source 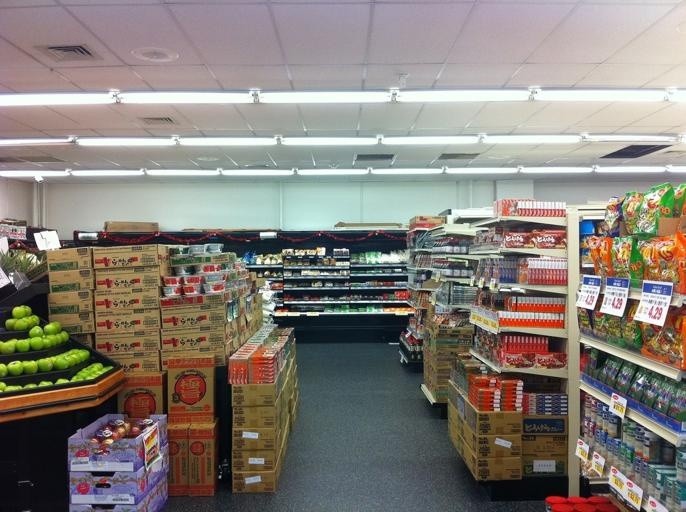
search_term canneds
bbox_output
[583,393,686,484]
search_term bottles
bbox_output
[397,331,425,362]
[414,270,428,289]
[428,235,476,279]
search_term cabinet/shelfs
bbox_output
[236,230,408,343]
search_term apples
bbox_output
[0,306,113,392]
[73,444,89,459]
[77,479,90,494]
[89,419,157,455]
